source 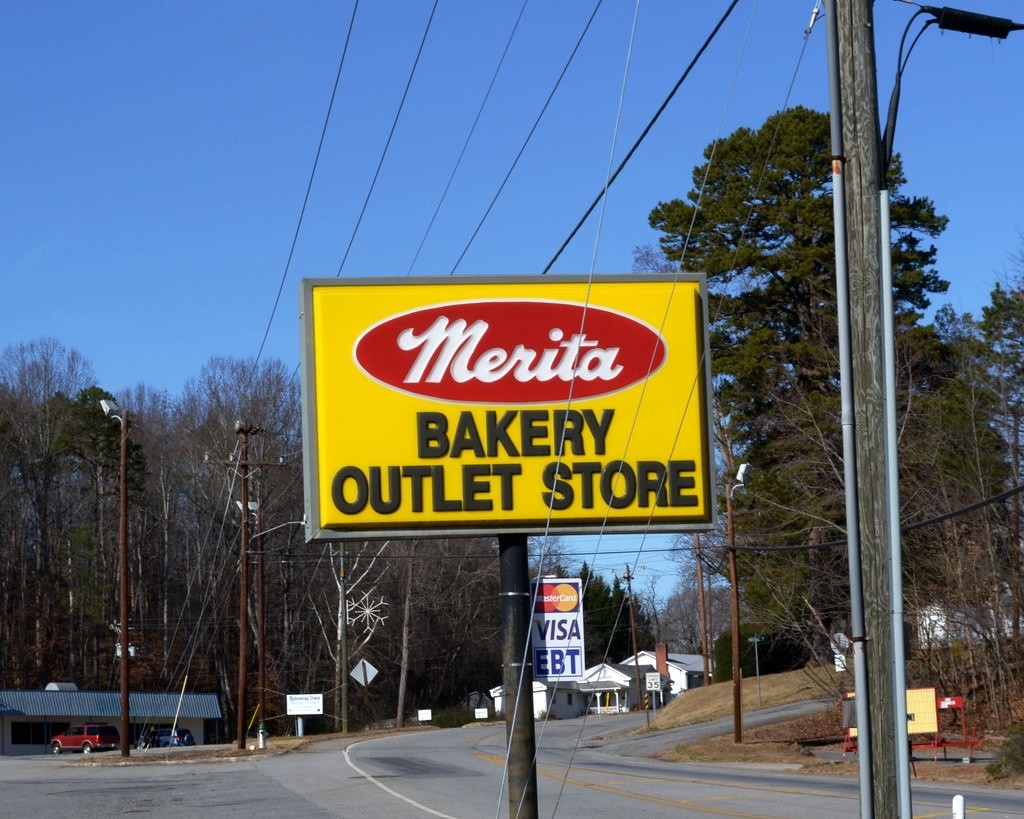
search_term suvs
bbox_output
[51,723,119,754]
[154,729,195,747]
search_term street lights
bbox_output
[100,399,129,757]
[725,463,752,742]
